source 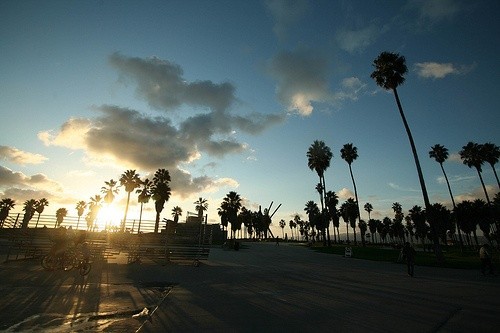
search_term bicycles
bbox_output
[41,239,94,277]
[125,236,285,268]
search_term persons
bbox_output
[274,236,281,247]
[402,241,416,277]
[430,245,436,254]
[480,242,490,275]
[487,244,499,274]
